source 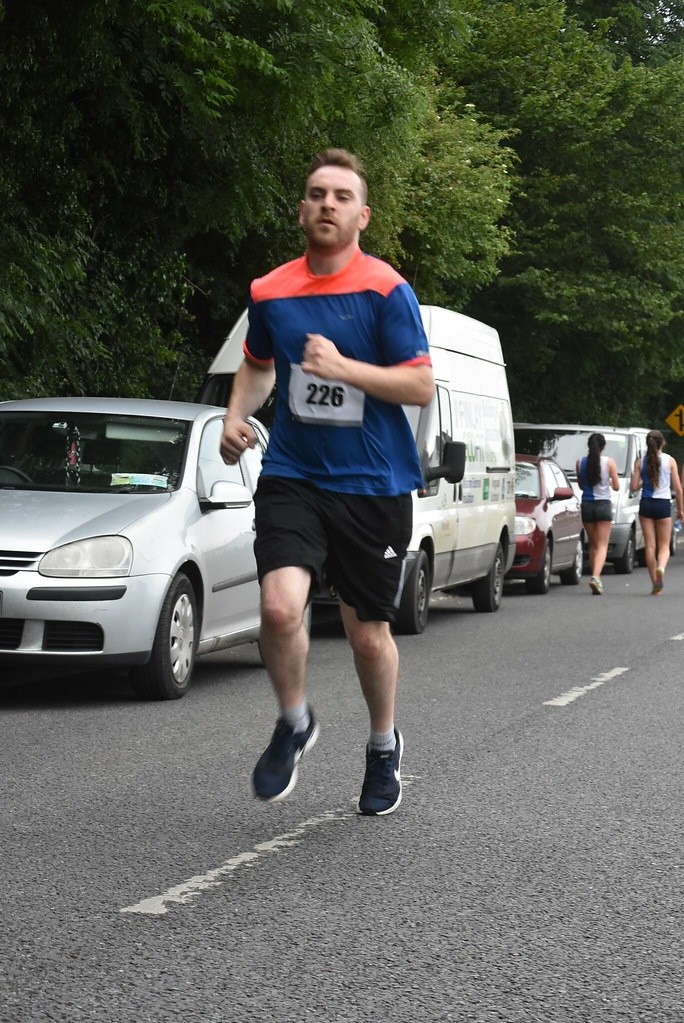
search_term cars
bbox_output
[504,452,589,595]
[0,396,313,700]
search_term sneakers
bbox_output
[652,584,662,596]
[356,729,405,816]
[250,700,319,803]
[656,568,664,589]
[592,590,598,595]
[589,576,603,595]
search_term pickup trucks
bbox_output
[511,421,681,575]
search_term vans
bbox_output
[193,303,516,635]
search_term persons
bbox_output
[631,430,684,595]
[221,149,436,815]
[576,433,619,595]
